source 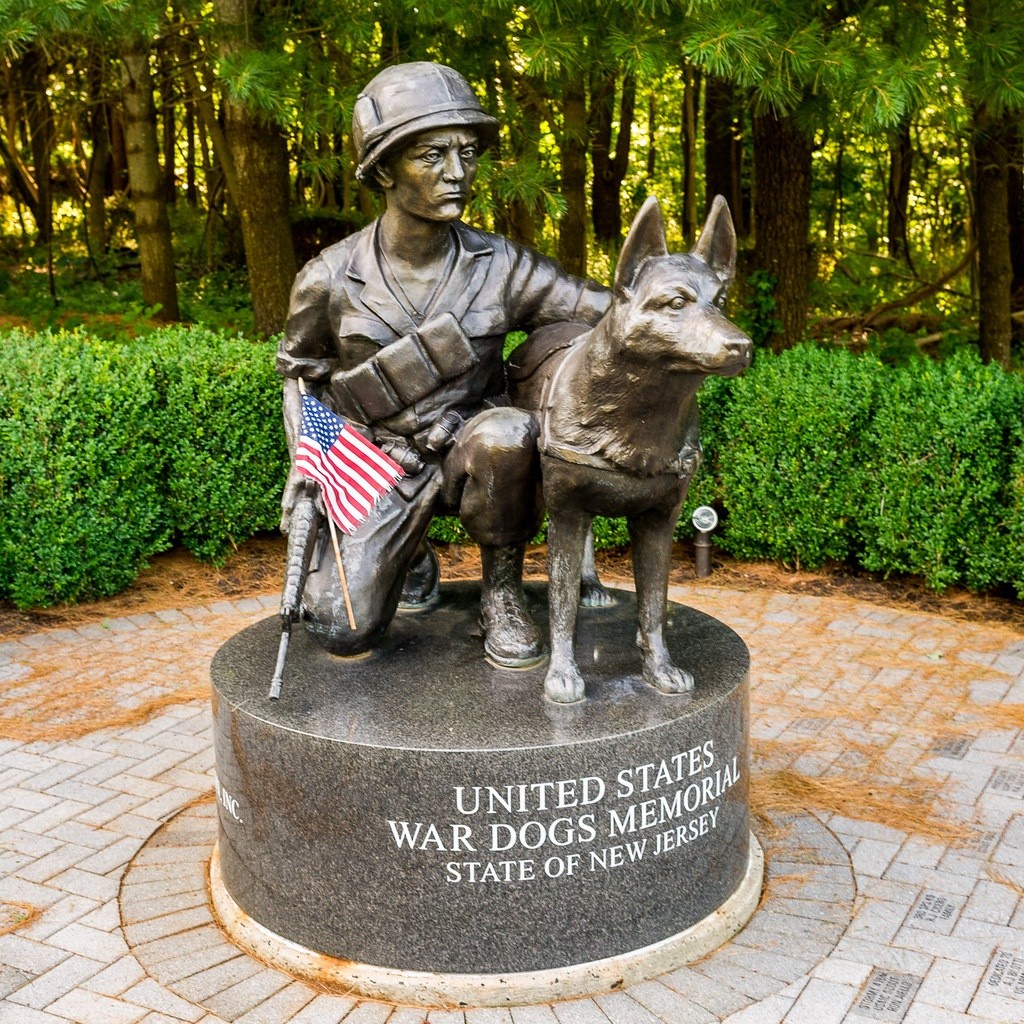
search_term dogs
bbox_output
[504,192,755,704]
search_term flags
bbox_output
[296,392,407,539]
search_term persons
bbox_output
[274,63,614,674]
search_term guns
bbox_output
[265,381,335,704]
